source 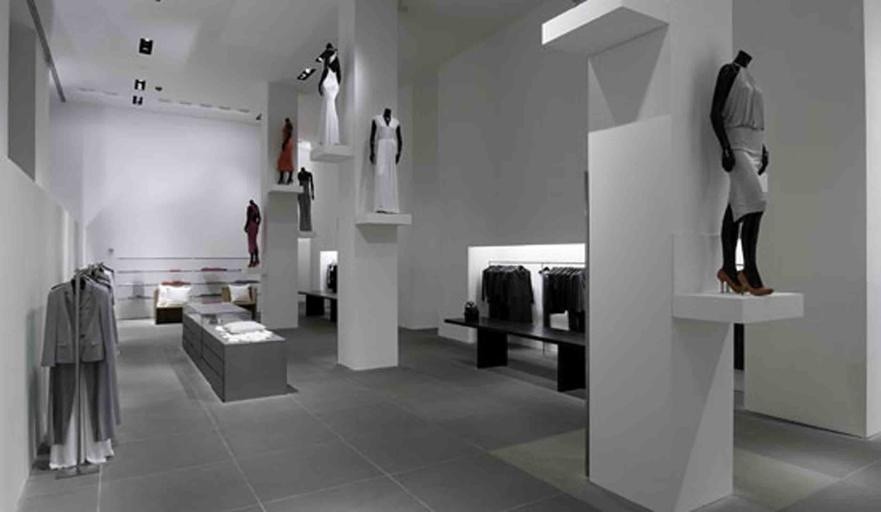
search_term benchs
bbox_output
[444,316,586,393]
[297,289,338,323]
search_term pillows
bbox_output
[156,282,169,308]
[166,284,191,307]
[228,283,251,303]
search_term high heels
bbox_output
[715,268,747,295]
[737,269,777,297]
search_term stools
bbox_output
[237,304,257,320]
[155,307,185,324]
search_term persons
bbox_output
[367,106,406,216]
[316,41,343,147]
[277,117,297,186]
[708,47,775,297]
[297,167,315,232]
[244,198,262,268]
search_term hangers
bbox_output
[51,261,116,293]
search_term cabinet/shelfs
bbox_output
[181,312,291,404]
[185,301,257,325]
